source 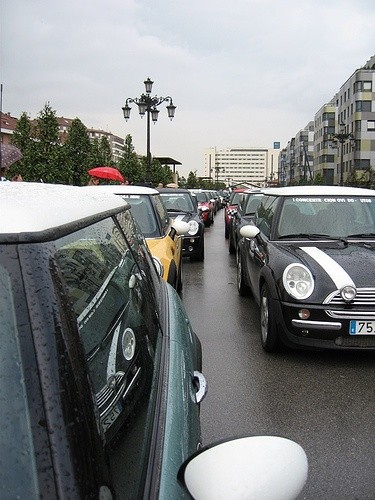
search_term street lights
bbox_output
[327,127,357,186]
[209,161,226,189]
[122,76,177,188]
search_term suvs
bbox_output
[0,178,207,500]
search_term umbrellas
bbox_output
[87,167,124,182]
[0,144,23,168]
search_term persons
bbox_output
[0,168,9,181]
[159,182,163,188]
[89,175,99,186]
[121,177,155,188]
[104,179,112,185]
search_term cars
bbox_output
[186,188,233,227]
[157,187,213,262]
[84,184,189,298]
[52,238,159,457]
[220,187,250,239]
[227,187,266,267]
[236,185,375,354]
